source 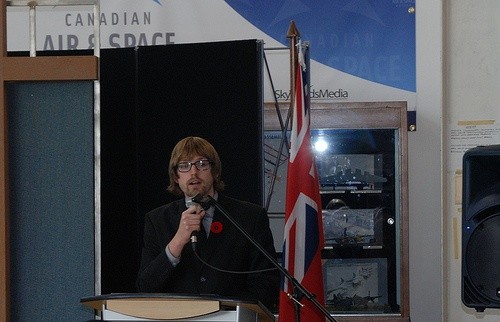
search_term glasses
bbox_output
[177,159,210,173]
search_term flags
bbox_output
[278,39,325,322]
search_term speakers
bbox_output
[462,144,500,312]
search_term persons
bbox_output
[135,135,280,318]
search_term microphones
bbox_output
[185,197,199,250]
